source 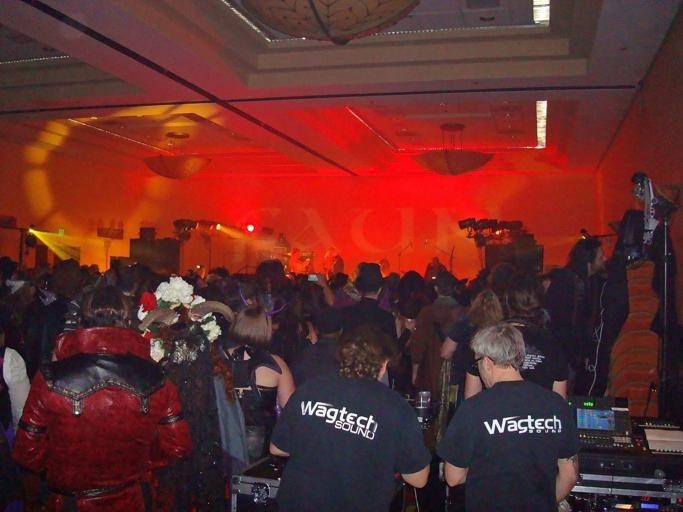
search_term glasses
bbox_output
[472,356,483,368]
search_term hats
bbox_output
[5,279,25,295]
[357,263,384,287]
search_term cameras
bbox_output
[307,274,319,281]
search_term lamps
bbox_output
[420,123,493,174]
[242,0,419,45]
[144,133,210,179]
